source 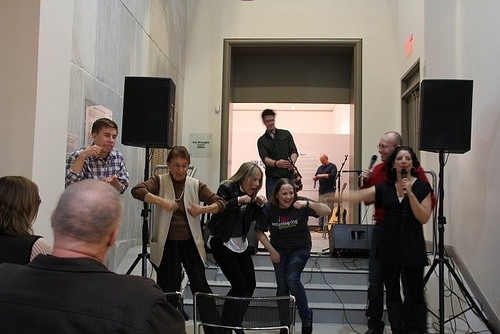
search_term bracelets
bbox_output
[305,200,309,208]
[273,160,277,168]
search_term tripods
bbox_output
[127,147,159,277]
[423,152,496,334]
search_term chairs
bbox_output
[193,291,296,334]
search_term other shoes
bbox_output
[314,227,322,232]
[362,326,384,334]
[279,319,292,334]
[301,309,313,334]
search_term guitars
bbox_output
[328,183,347,231]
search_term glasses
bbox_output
[169,162,189,171]
[264,119,274,123]
[377,144,396,149]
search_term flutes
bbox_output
[271,130,302,178]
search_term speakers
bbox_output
[417,79,473,153]
[329,224,374,258]
[121,77,175,149]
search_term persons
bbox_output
[0,176,52,265]
[0,179,186,334]
[131,145,227,334]
[256,178,332,334]
[65,118,129,195]
[257,109,298,200]
[322,132,436,334]
[204,162,268,334]
[313,154,338,232]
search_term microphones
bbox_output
[401,169,407,193]
[368,155,378,169]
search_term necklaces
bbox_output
[175,192,184,201]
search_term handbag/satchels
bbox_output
[291,169,304,193]
